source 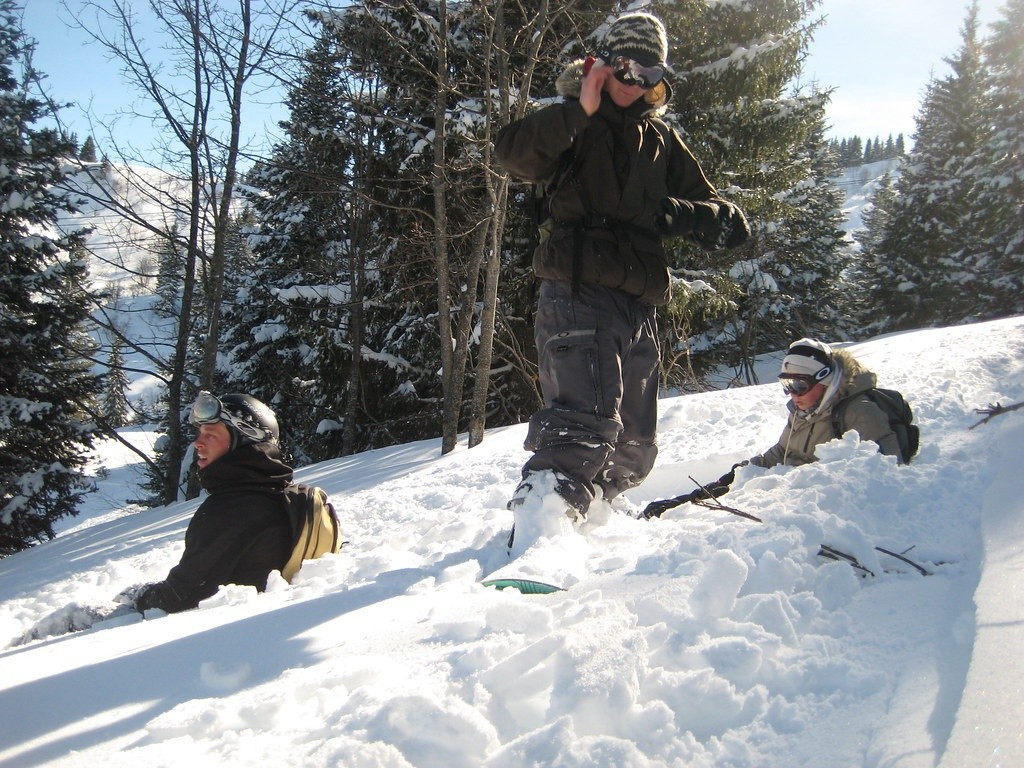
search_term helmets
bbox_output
[218,393,280,453]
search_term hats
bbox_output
[602,13,667,65]
[782,338,834,386]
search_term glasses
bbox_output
[777,373,813,395]
[189,391,224,426]
[610,54,664,89]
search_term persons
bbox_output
[493,14,750,548]
[6,391,294,647]
[644,339,905,520]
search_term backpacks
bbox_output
[832,387,920,464]
[218,482,340,588]
